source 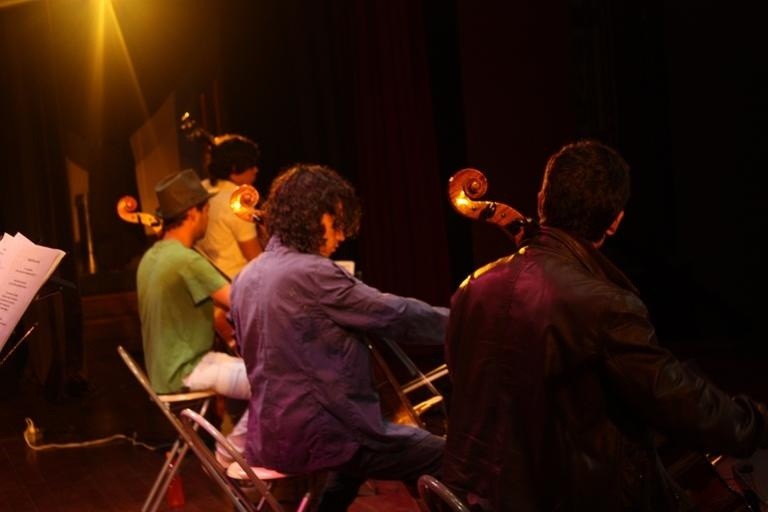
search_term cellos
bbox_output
[116,194,249,418]
[231,184,428,430]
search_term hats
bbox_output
[154,168,220,218]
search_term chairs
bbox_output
[113,344,333,512]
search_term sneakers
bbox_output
[215,444,288,480]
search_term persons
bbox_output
[136,169,253,468]
[230,161,451,512]
[200,132,263,281]
